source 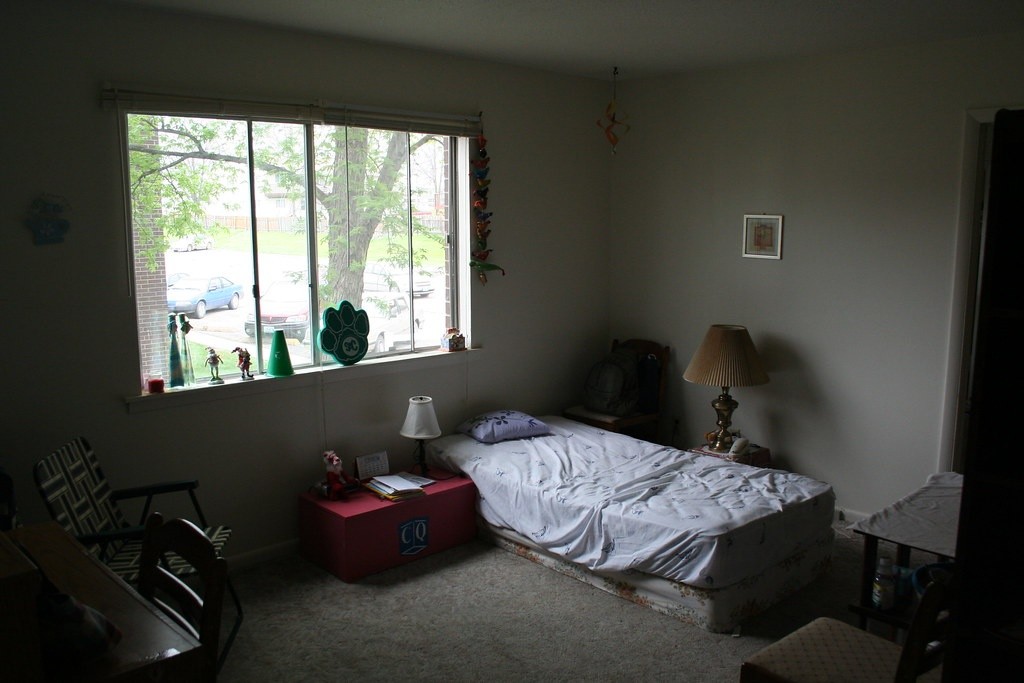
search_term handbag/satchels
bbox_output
[583,346,661,417]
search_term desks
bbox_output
[0,516,204,683]
[845,473,963,631]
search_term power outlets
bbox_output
[672,416,681,430]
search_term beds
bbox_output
[423,414,836,633]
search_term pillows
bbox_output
[455,410,549,441]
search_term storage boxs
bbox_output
[297,464,476,583]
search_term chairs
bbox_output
[564,338,670,441]
[740,557,994,683]
[35,436,243,675]
[138,511,228,683]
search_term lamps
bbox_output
[683,324,769,453]
[400,395,441,477]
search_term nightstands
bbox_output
[688,444,771,468]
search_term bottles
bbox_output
[267,329,294,376]
[872,556,896,612]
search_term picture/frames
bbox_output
[742,215,782,259]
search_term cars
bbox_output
[361,291,419,355]
[245,289,310,343]
[166,276,242,319]
[171,232,213,252]
[362,261,435,296]
